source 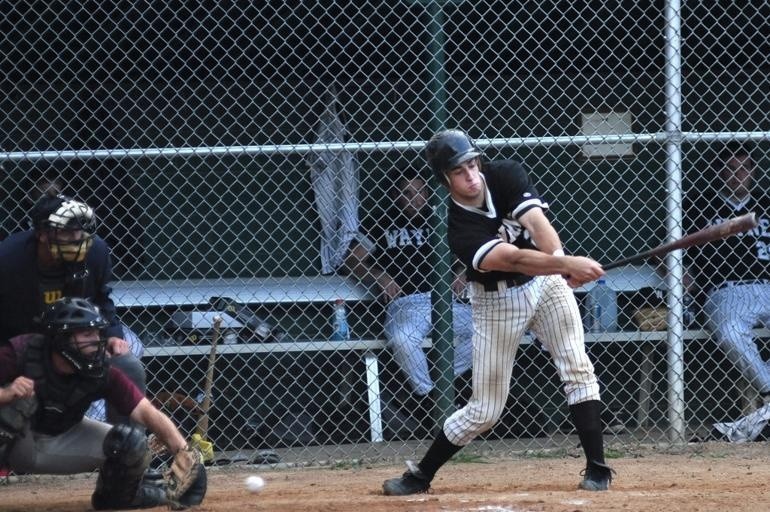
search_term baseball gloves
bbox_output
[162,448,207,508]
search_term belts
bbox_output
[714,280,764,291]
[484,277,533,291]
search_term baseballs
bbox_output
[244,476,263,493]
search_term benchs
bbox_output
[108,264,770,451]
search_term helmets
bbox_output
[41,296,110,345]
[33,197,96,232]
[426,129,481,186]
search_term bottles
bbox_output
[591,304,600,332]
[333,298,346,339]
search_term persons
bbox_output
[382,130,617,496]
[1,181,165,486]
[1,298,207,511]
[682,140,770,441]
[344,164,474,439]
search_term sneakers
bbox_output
[577,460,618,489]
[382,461,430,495]
[91,483,168,510]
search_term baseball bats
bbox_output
[194,317,223,441]
[561,212,759,280]
